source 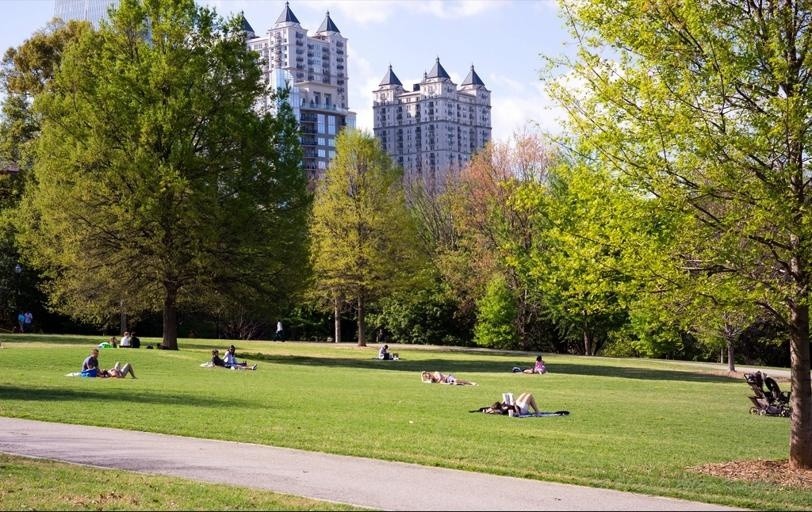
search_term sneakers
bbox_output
[252,364,257,370]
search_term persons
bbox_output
[24,310,33,332]
[97,362,137,379]
[222,345,237,364]
[378,345,393,360]
[491,392,544,416]
[533,356,546,375]
[110,332,140,348]
[18,311,25,334]
[421,371,476,385]
[275,319,285,342]
[82,349,100,374]
[211,350,257,370]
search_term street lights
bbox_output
[13,263,23,331]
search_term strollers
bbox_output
[743,371,792,419]
